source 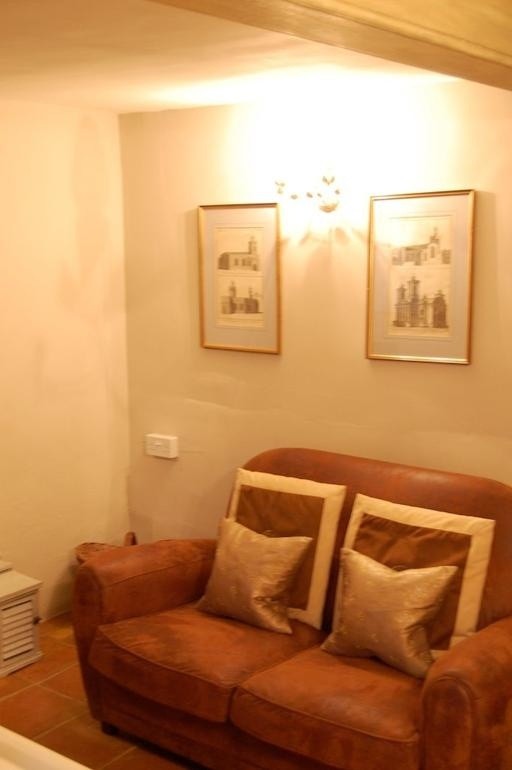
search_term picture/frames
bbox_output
[363,188,473,367]
[196,202,284,359]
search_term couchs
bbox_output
[71,447,512,769]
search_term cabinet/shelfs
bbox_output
[1,563,47,683]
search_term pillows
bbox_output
[318,545,459,677]
[195,513,315,636]
